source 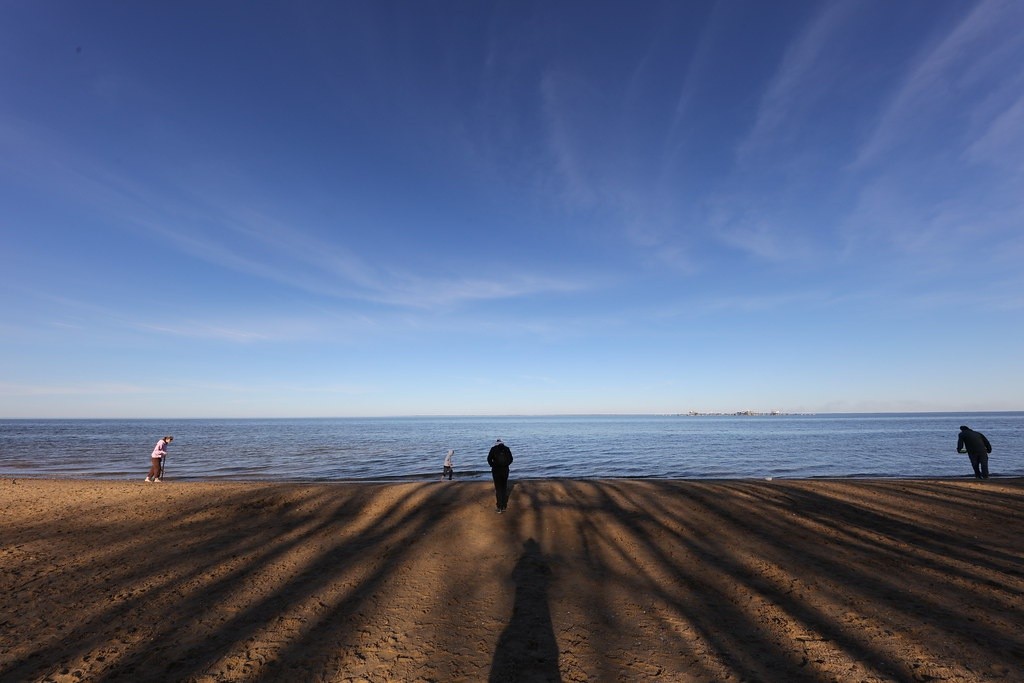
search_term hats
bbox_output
[495,439,504,445]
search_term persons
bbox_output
[957,426,992,480]
[145,435,173,482]
[487,438,513,513]
[440,449,454,481]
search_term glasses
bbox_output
[169,438,172,440]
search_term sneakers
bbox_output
[145,478,151,482]
[494,507,506,514]
[154,478,161,482]
